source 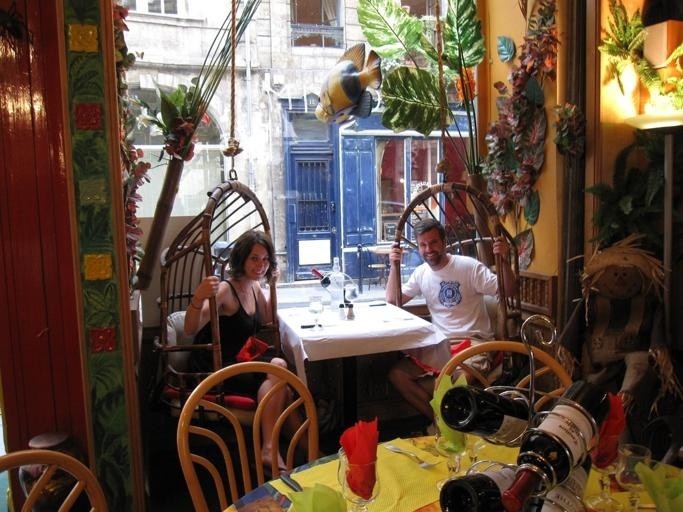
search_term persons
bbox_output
[385,219,518,436]
[555,233,681,418]
[184,230,328,471]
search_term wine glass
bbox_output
[614,444,652,512]
[308,296,324,326]
[584,434,625,512]
[337,447,380,512]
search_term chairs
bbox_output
[149,169,279,448]
[395,172,528,386]
[176,361,319,512]
[434,340,575,437]
[0,449,109,512]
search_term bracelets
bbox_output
[190,300,203,310]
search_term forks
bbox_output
[384,442,445,469]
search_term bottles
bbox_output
[311,268,351,303]
[502,380,610,510]
[347,304,355,319]
[338,304,346,319]
[439,465,517,512]
[440,385,529,445]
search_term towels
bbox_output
[590,393,627,467]
[339,416,379,500]
[287,483,346,512]
[429,374,468,453]
[634,460,683,512]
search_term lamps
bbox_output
[637,0,683,130]
